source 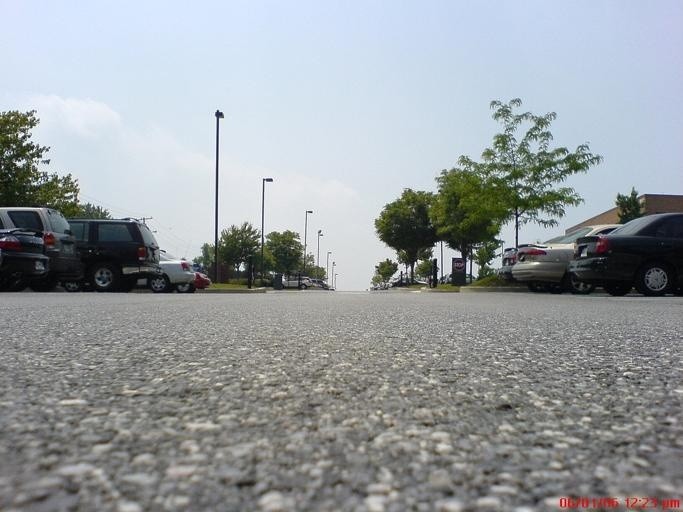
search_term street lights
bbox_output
[213,108,223,284]
[259,176,339,291]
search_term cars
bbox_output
[370,269,478,287]
[511,223,624,294]
[498,247,548,293]
[565,210,681,298]
[0,206,213,293]
